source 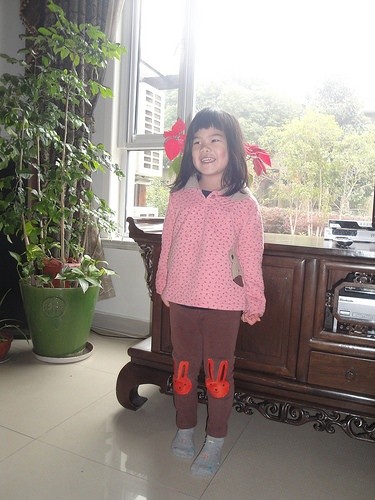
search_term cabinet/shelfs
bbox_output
[116,212,375,445]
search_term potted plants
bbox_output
[0,0,129,366]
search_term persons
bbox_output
[155,106,266,477]
